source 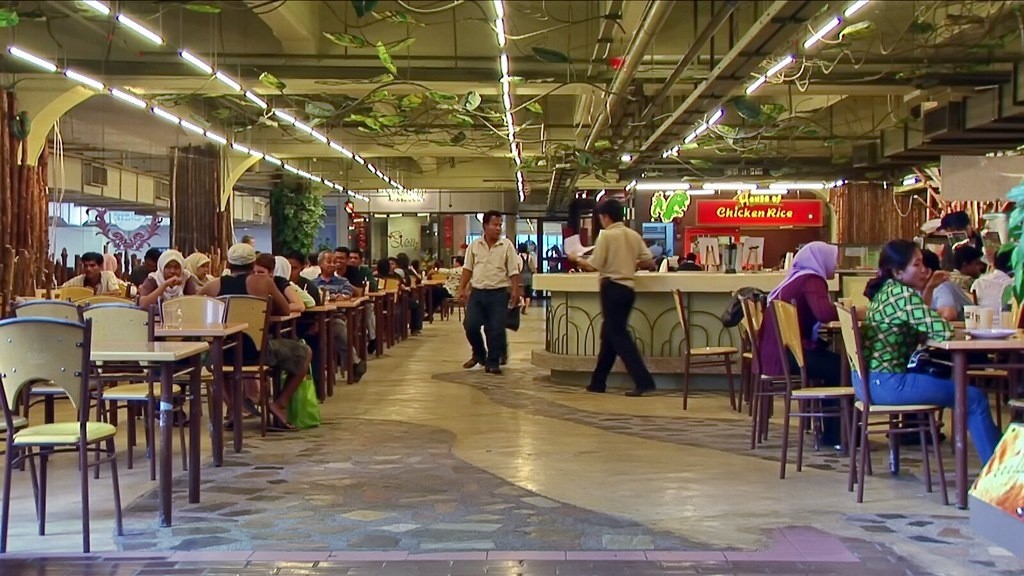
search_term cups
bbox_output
[303,290,307,293]
[963,305,994,331]
[364,281,370,293]
[838,298,852,309]
[784,251,793,270]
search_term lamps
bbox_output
[4,0,873,201]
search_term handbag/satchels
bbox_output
[280,339,320,429]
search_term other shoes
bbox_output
[587,385,606,393]
[424,316,434,322]
[824,430,861,450]
[354,361,367,382]
[410,329,421,336]
[155,411,190,427]
[463,355,486,368]
[900,432,947,445]
[625,385,656,396]
[485,363,502,374]
[367,338,376,354]
[224,420,233,430]
[242,409,254,421]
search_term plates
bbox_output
[963,328,1017,338]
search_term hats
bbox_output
[227,243,257,265]
[936,211,971,231]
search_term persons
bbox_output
[96,235,704,418]
[137,249,196,307]
[851,238,1003,466]
[196,242,312,431]
[898,211,1014,445]
[751,240,878,451]
[568,200,657,396]
[459,210,520,373]
[61,252,122,295]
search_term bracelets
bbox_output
[575,256,582,266]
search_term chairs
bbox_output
[0,280,465,554]
[673,288,1024,509]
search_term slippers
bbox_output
[265,423,300,431]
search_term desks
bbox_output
[89,341,210,528]
[267,312,300,425]
[921,329,1024,509]
[325,299,361,384]
[305,306,337,403]
[153,320,249,467]
[346,295,370,375]
[817,320,862,457]
[365,291,387,358]
